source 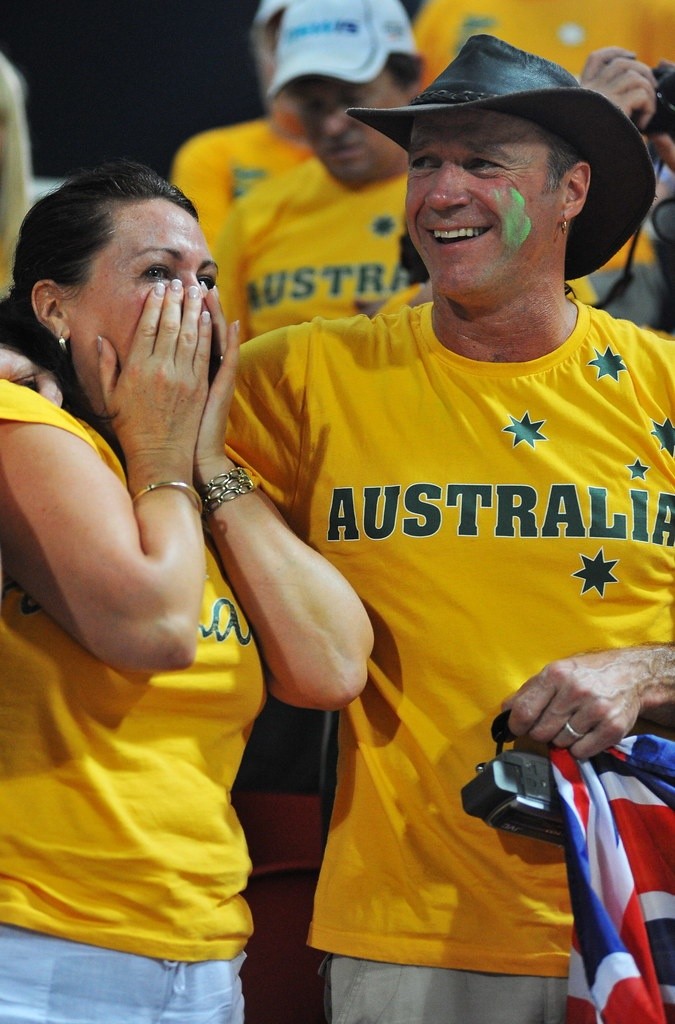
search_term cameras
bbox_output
[629,63,675,138]
[461,749,566,846]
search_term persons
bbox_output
[0,165,375,1024]
[0,34,675,1024]
[167,0,675,345]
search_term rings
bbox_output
[565,721,584,739]
[211,353,224,365]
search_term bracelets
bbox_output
[133,481,202,516]
[202,466,255,518]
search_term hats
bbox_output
[344,35,655,280]
[266,0,415,97]
